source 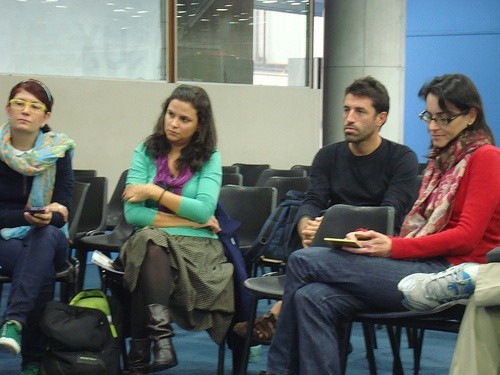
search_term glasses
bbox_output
[419,108,470,126]
[8,99,49,115]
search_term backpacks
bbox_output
[239,187,313,285]
[38,287,122,375]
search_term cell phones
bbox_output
[24,208,45,214]
[323,237,362,250]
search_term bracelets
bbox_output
[156,188,167,203]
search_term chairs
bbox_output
[0,164,499,374]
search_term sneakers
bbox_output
[233,313,280,347]
[397,259,481,316]
[22,363,40,375]
[0,320,23,357]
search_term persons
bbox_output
[396,248,499,374]
[232,75,418,345]
[118,83,238,375]
[1,78,78,375]
[264,75,500,375]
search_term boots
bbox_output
[123,338,150,374]
[143,302,180,371]
